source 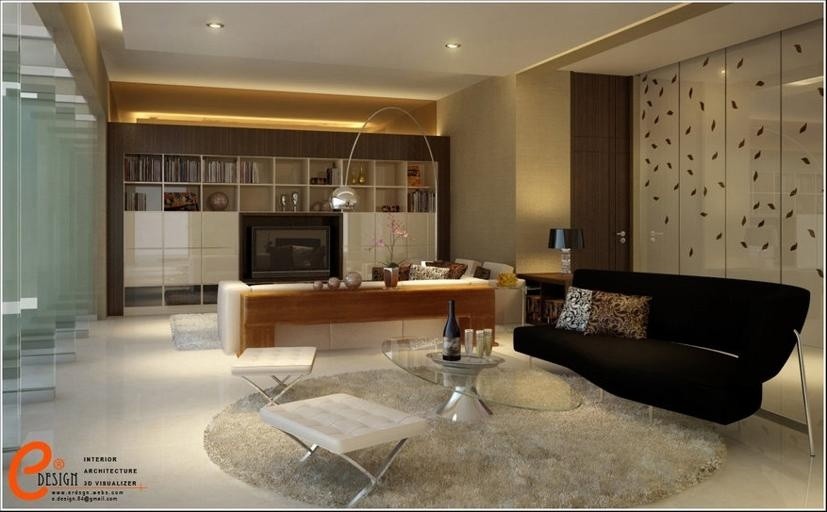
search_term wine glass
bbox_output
[475,330,485,364]
[280,195,287,212]
[482,329,493,362]
[291,192,299,212]
[464,329,474,362]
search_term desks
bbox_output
[240,283,501,358]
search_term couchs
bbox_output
[506,263,819,458]
[214,274,483,355]
[407,252,526,329]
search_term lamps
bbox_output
[546,225,585,276]
[325,105,441,265]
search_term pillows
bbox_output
[580,286,654,344]
[426,257,470,279]
[407,259,450,282]
[472,264,491,281]
[552,285,597,333]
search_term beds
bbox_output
[374,333,584,429]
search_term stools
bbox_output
[229,344,319,409]
[256,391,432,509]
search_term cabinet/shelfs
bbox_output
[119,152,240,316]
[515,269,575,328]
[234,153,335,215]
[333,156,440,282]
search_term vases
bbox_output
[380,264,403,289]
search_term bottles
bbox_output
[350,175,357,185]
[442,299,461,361]
[358,162,367,185]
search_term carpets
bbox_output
[193,357,738,511]
[167,310,225,352]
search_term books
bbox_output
[126,191,147,211]
[240,160,258,183]
[408,166,422,186]
[326,168,338,185]
[408,189,435,212]
[125,156,161,182]
[203,159,237,183]
[164,157,201,182]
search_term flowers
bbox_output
[356,211,417,269]
